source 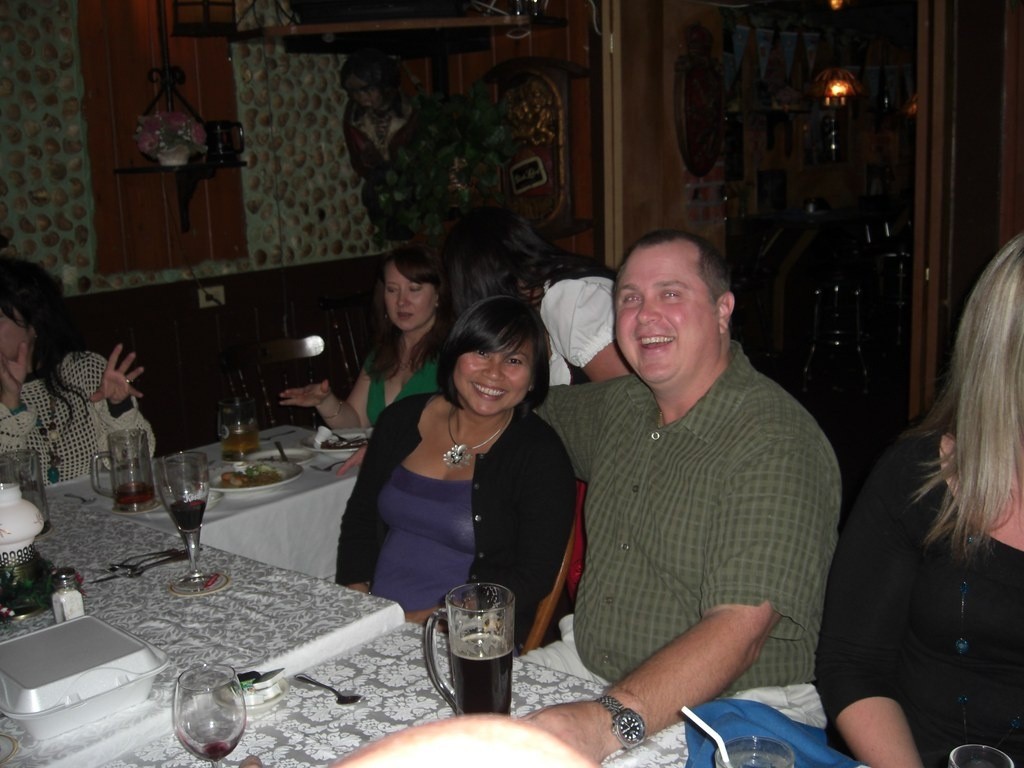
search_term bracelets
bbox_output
[322,401,342,419]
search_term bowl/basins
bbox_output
[0,614,170,742]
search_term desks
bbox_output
[0,427,872,768]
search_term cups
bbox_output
[948,744,1015,768]
[423,582,516,718]
[91,429,156,512]
[0,449,53,537]
[714,736,795,768]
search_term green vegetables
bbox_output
[245,466,281,477]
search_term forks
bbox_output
[79,547,204,578]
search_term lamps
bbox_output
[808,66,871,107]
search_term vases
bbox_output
[154,144,193,168]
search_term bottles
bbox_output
[51,567,85,624]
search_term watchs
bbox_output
[594,694,647,749]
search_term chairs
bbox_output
[215,287,380,436]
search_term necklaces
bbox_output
[955,534,1024,765]
[442,404,511,471]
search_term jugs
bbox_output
[217,395,261,461]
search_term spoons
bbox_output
[294,673,366,707]
[308,460,347,472]
[108,549,187,571]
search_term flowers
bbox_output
[131,109,210,153]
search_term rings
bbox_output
[125,378,134,385]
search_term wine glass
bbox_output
[171,663,247,768]
[154,451,229,595]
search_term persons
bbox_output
[531,228,843,768]
[335,292,578,657]
[0,258,155,486]
[815,233,1024,768]
[280,212,636,477]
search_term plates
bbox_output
[243,447,315,466]
[209,458,304,499]
[212,670,289,716]
[298,427,375,460]
